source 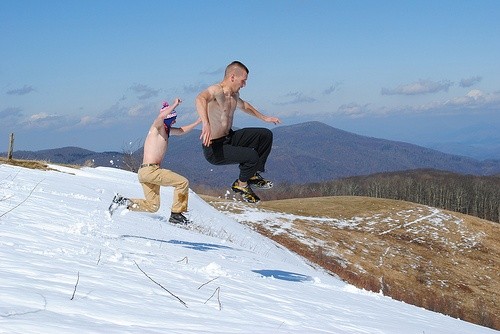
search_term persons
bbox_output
[195,61,281,205]
[109,98,203,226]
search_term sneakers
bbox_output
[231,178,261,203]
[248,173,273,190]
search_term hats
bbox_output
[161,101,177,138]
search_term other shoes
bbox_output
[169,214,193,225]
[108,193,123,216]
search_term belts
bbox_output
[141,163,160,168]
